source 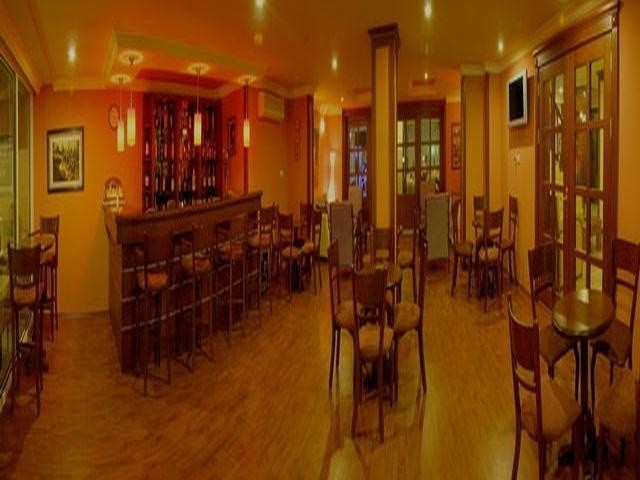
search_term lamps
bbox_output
[112,50,256,151]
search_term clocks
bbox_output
[109,104,120,129]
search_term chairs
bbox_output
[7,215,59,417]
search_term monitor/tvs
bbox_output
[505,67,528,128]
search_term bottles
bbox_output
[145,99,218,212]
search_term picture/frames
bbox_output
[47,126,84,193]
[451,122,462,170]
